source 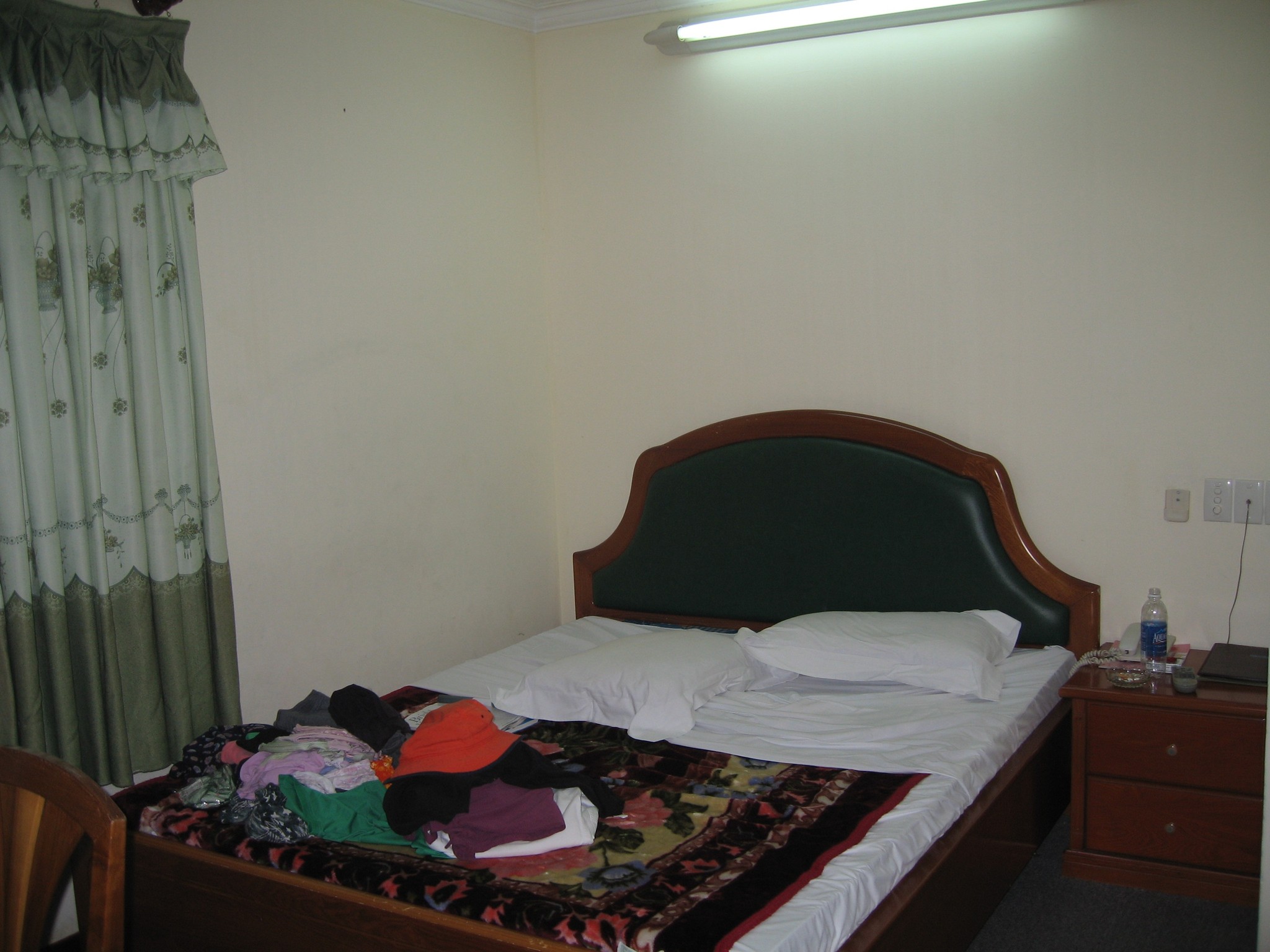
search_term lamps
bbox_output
[642,0,1083,58]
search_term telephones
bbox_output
[1115,621,1178,662]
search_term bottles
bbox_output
[1140,588,1168,685]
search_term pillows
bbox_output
[733,609,1021,702]
[486,628,800,742]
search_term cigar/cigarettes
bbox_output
[1118,675,1140,682]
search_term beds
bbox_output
[76,410,1102,952]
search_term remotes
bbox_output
[1171,665,1197,693]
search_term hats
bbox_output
[382,699,522,784]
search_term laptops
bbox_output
[1198,642,1269,687]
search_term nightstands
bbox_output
[1056,642,1269,911]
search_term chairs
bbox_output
[0,744,126,952]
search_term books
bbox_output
[1098,640,1190,673]
